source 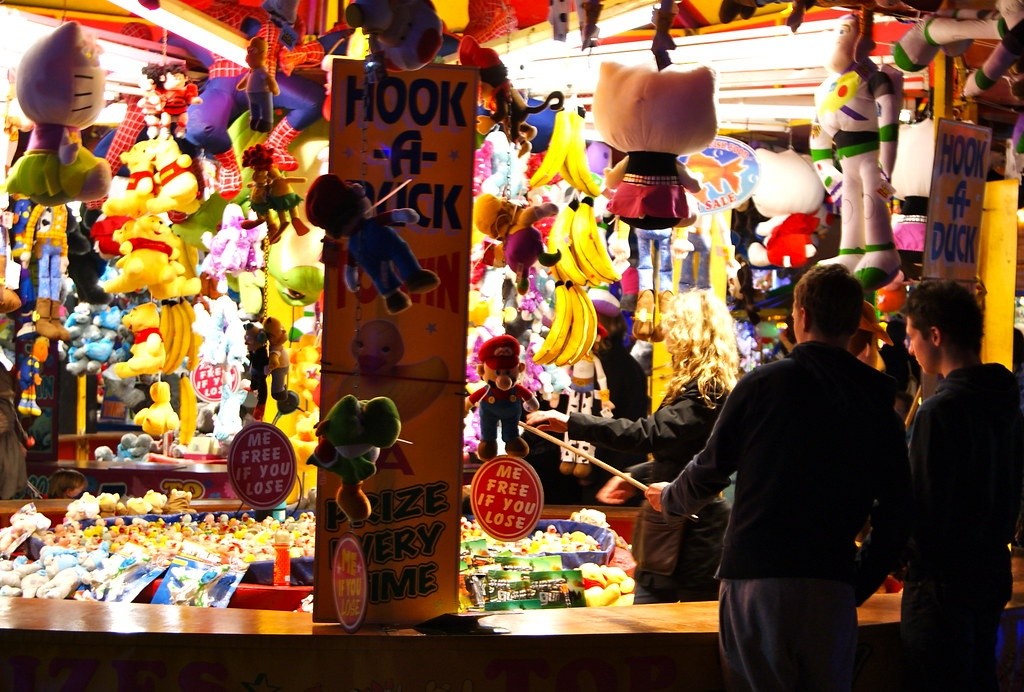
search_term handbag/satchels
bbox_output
[631,504,685,576]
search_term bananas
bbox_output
[529,109,623,369]
[159,297,202,446]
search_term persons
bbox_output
[850,282,1024,692]
[845,300,880,372]
[645,263,910,692]
[526,289,738,605]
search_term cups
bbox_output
[76,435,90,469]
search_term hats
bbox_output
[858,300,894,346]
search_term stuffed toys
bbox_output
[0,0,1024,531]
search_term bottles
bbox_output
[271,543,291,587]
